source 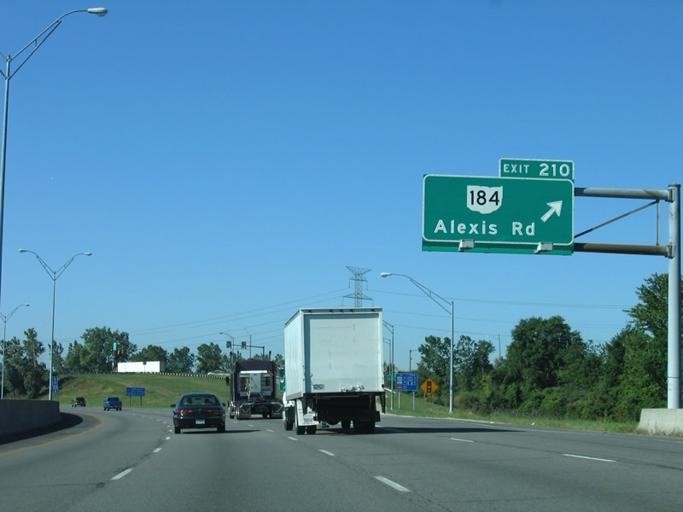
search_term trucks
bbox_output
[226,359,283,420]
[282,307,385,434]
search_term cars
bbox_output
[170,394,226,433]
[72,397,86,407]
[103,397,122,411]
[207,370,230,377]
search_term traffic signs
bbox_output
[421,176,576,245]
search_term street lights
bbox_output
[0,304,29,400]
[381,272,454,414]
[0,7,107,298]
[219,332,234,353]
[18,249,92,401]
